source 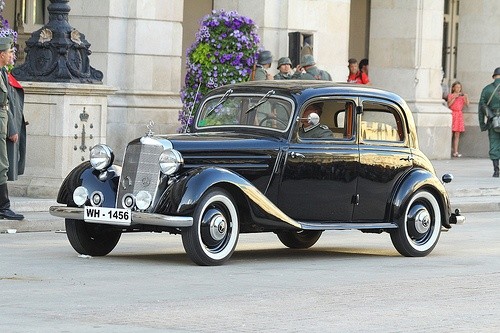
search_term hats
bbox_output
[0,38,12,51]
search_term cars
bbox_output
[49,80,465,266]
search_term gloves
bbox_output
[480,123,486,132]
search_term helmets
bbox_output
[493,67,500,78]
[313,102,323,116]
[258,51,272,64]
[300,55,314,67]
[277,58,292,69]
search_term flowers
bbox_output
[176,8,264,134]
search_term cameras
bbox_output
[459,94,464,96]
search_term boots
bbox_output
[0,184,24,220]
[493,159,499,177]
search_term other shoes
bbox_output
[453,152,463,157]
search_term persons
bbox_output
[447,82,469,158]
[478,68,500,177]
[0,37,27,220]
[247,50,371,137]
